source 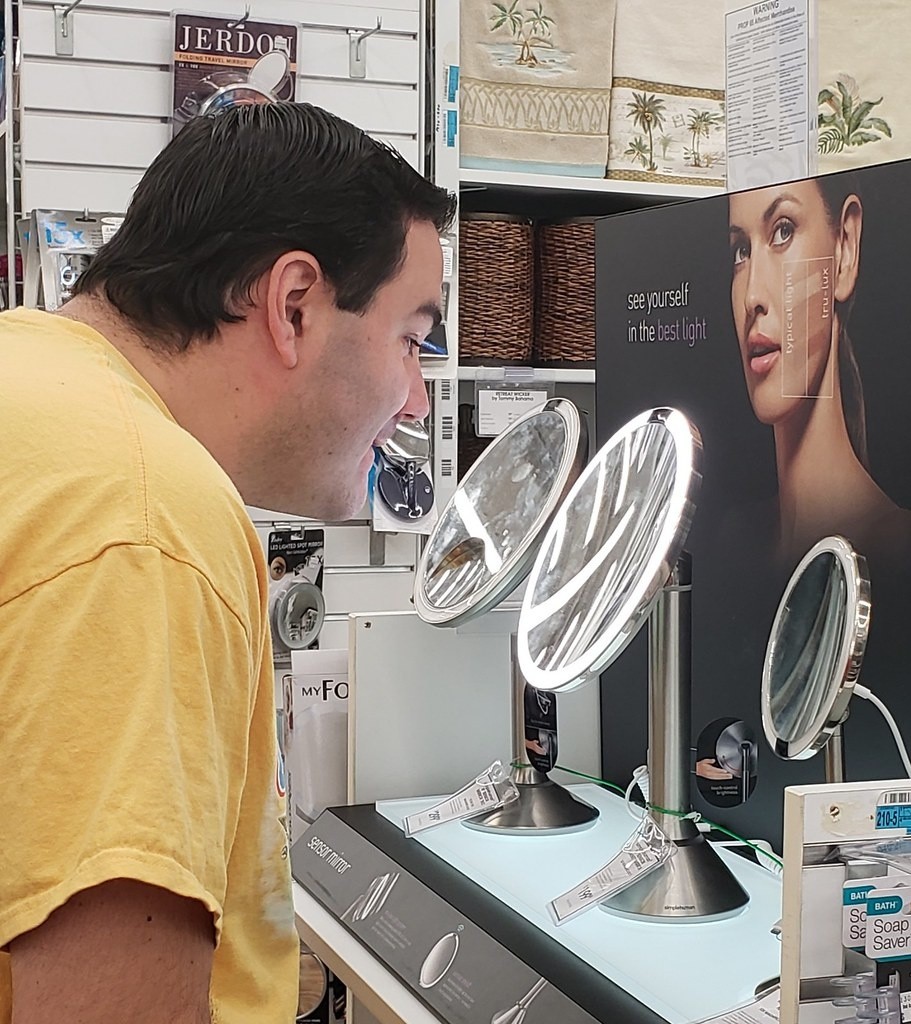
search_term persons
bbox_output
[0,101,458,1024]
[697,757,734,781]
[731,173,911,587]
[526,739,547,757]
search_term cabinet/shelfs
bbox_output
[419,1,725,383]
